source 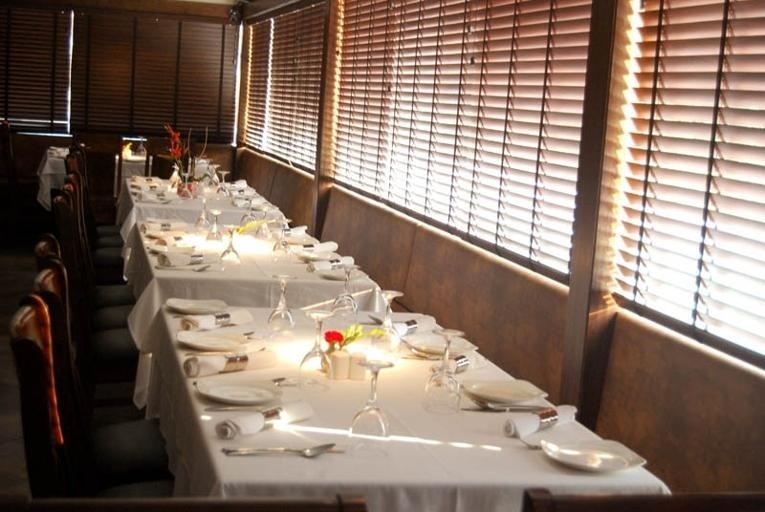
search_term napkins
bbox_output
[446,351,489,374]
[391,311,438,335]
[181,345,276,377]
[184,305,254,332]
[502,402,578,438]
[214,398,313,447]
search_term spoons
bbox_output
[222,443,337,459]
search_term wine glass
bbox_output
[266,273,299,335]
[194,197,243,269]
[296,309,335,394]
[347,357,396,451]
[238,196,293,267]
[329,262,361,325]
[178,171,193,201]
[421,328,465,416]
[375,288,404,354]
[208,164,231,200]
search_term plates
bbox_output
[291,245,342,262]
[150,243,203,258]
[175,328,251,353]
[539,437,647,474]
[401,332,479,360]
[460,376,548,407]
[195,372,282,406]
[165,297,230,316]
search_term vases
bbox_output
[321,345,339,373]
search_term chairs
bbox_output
[9,292,176,480]
[29,255,154,423]
[518,485,764,512]
[38,139,390,331]
[32,231,144,377]
[19,480,370,511]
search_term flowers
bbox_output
[324,323,365,349]
